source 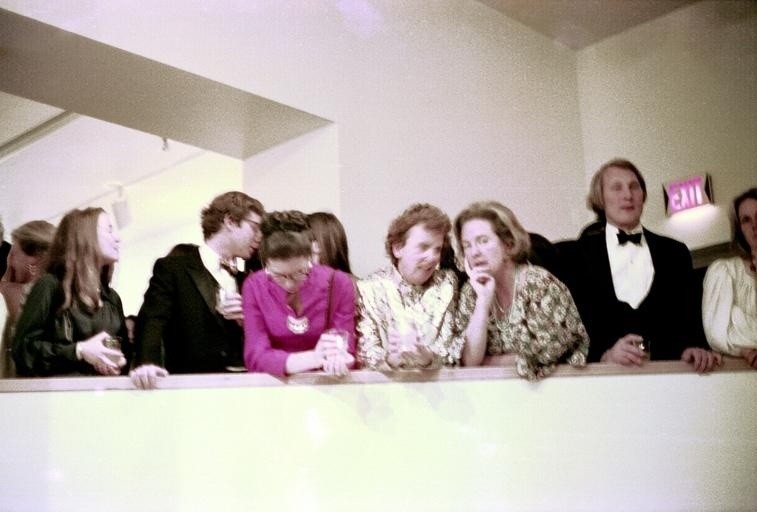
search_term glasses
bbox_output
[242,215,267,234]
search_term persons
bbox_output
[356,204,463,373]
[13,202,127,380]
[703,187,756,367]
[304,209,350,272]
[0,221,56,316]
[451,200,584,374]
[245,211,362,377]
[568,158,724,374]
[129,188,265,388]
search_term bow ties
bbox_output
[615,227,643,245]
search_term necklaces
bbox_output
[494,290,509,321]
